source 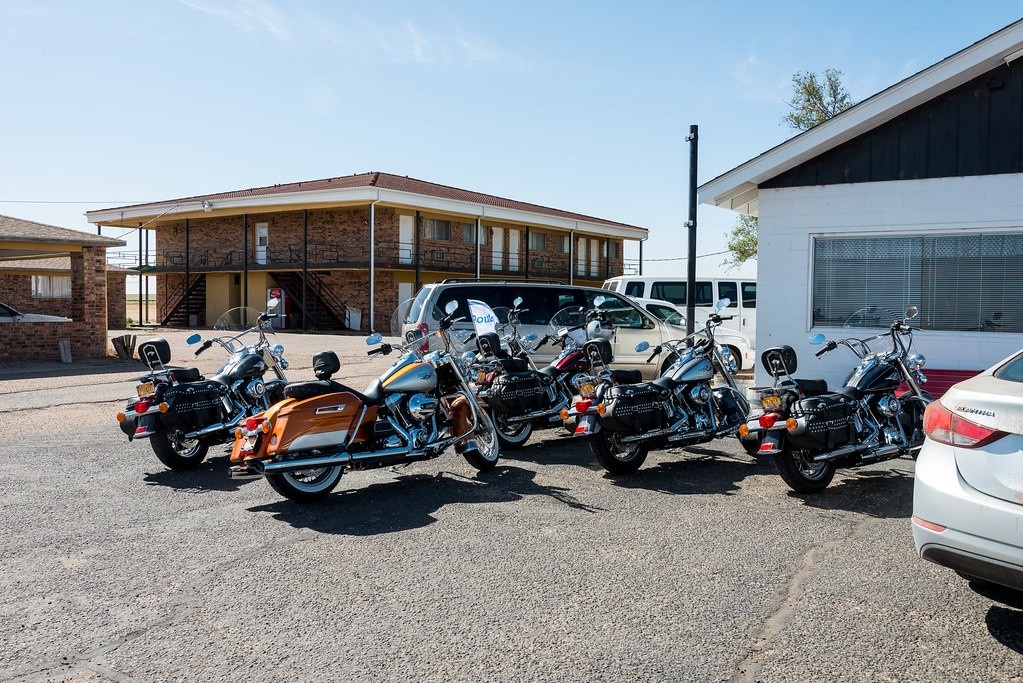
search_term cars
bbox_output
[912,347,1023,611]
[0,301,73,322]
[626,299,755,376]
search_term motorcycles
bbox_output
[738,302,938,493]
[466,296,621,452]
[225,298,500,501]
[111,299,304,468]
[556,299,764,480]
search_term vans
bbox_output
[599,276,758,358]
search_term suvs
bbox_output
[404,277,724,391]
